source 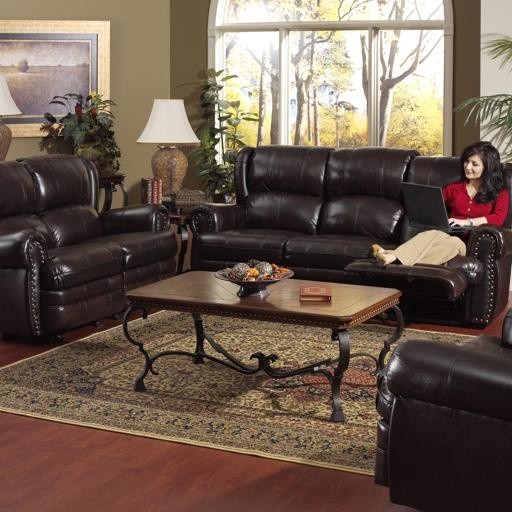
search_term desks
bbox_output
[159,206,193,275]
[92,173,128,216]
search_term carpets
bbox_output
[0,306,478,481]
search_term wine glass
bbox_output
[215,266,295,298]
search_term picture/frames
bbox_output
[0,32,100,125]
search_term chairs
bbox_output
[372,306,512,511]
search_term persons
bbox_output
[371,142,510,268]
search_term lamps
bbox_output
[136,97,204,196]
[0,72,24,161]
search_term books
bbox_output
[141,177,163,205]
[299,284,332,305]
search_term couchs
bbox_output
[0,152,180,346]
[188,145,512,330]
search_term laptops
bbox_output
[400,181,478,234]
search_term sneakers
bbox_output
[368,242,382,258]
[375,252,387,268]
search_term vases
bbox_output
[76,144,105,170]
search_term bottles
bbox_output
[141,177,163,205]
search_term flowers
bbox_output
[36,89,123,175]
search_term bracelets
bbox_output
[468,217,473,227]
